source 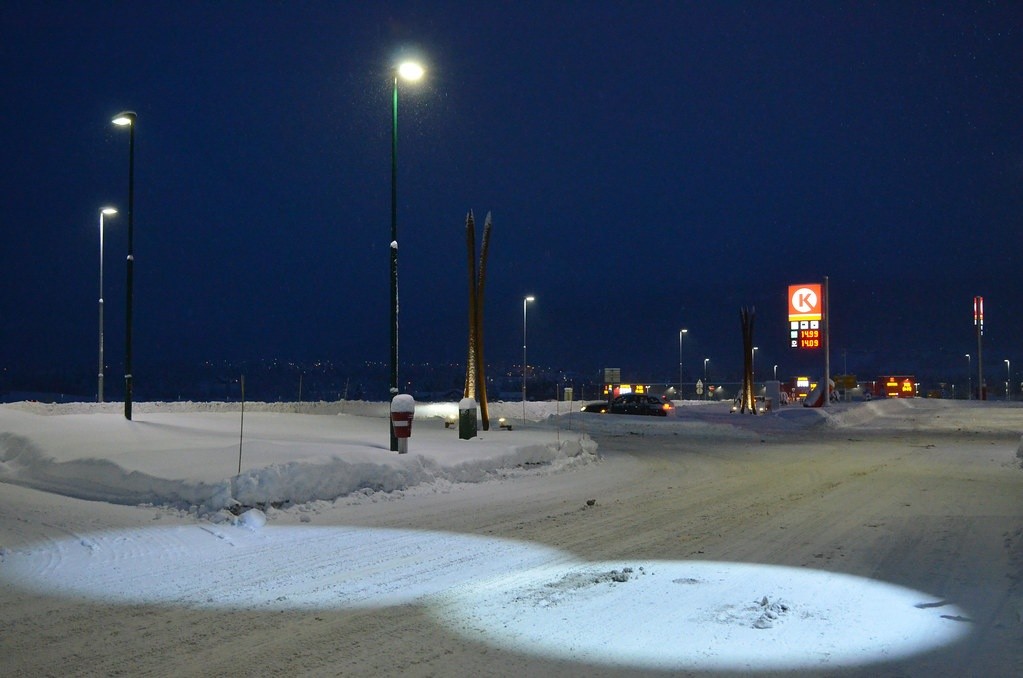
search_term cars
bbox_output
[579,392,676,417]
[729,395,766,415]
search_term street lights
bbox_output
[773,365,778,380]
[1004,360,1011,404]
[964,354,971,400]
[704,358,710,400]
[522,296,535,425]
[387,59,425,452]
[679,329,688,401]
[98,206,120,404]
[752,346,759,386]
[110,111,139,422]
